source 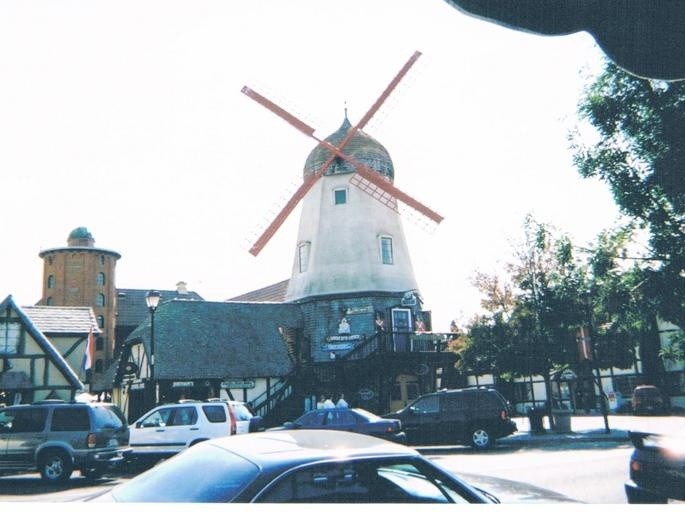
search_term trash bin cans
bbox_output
[553,410,573,433]
[527,407,545,433]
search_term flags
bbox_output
[81,326,95,372]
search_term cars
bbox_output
[624,429,685,504]
[631,385,664,416]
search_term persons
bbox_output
[335,393,349,423]
[450,319,459,338]
[317,395,326,419]
[324,392,339,423]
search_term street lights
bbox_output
[580,290,611,433]
[144,290,161,406]
